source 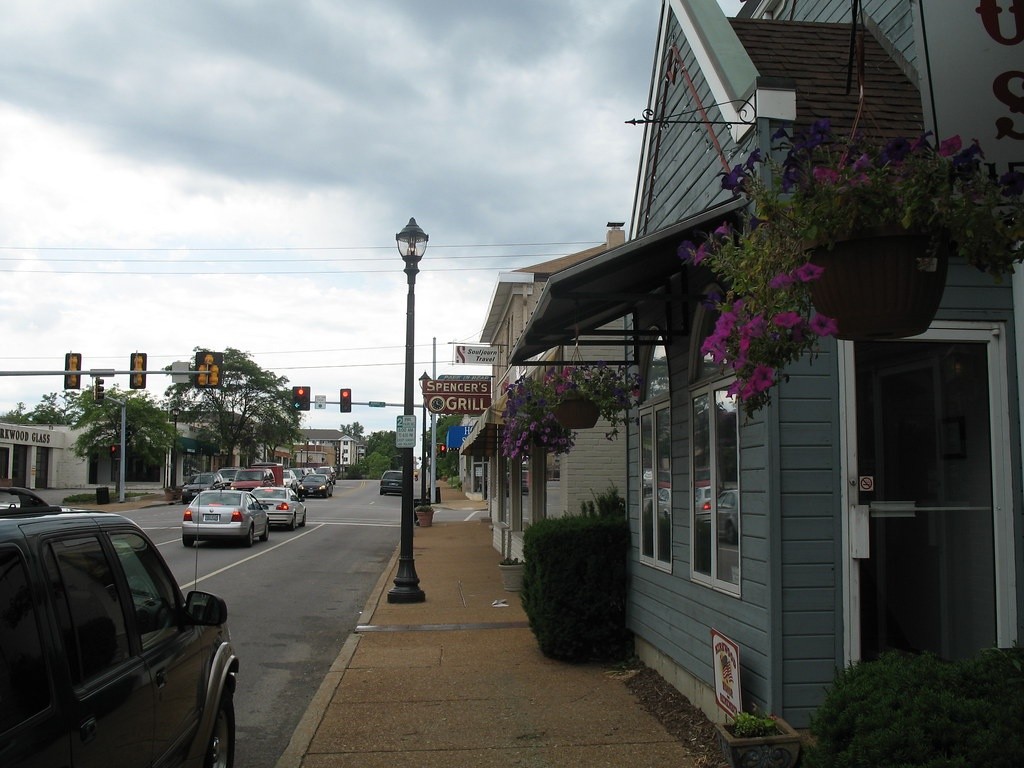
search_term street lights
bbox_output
[419,371,432,507]
[306,437,310,468]
[388,216,429,603]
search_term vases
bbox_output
[549,397,600,430]
[799,223,952,343]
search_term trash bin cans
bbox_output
[428,487,441,504]
[414,498,430,522]
[97,488,109,504]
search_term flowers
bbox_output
[498,360,644,466]
[674,115,1024,428]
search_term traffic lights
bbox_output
[441,444,447,455]
[95,375,105,401]
[340,388,352,413]
[292,386,310,411]
[171,407,180,487]
[109,444,117,459]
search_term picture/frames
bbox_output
[940,417,966,461]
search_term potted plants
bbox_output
[713,712,804,768]
[499,557,526,593]
[164,486,182,501]
[414,505,434,527]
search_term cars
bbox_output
[297,474,334,498]
[315,466,336,484]
[380,470,403,495]
[181,489,270,547]
[181,462,316,504]
[250,486,307,531]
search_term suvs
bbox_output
[0,483,239,768]
